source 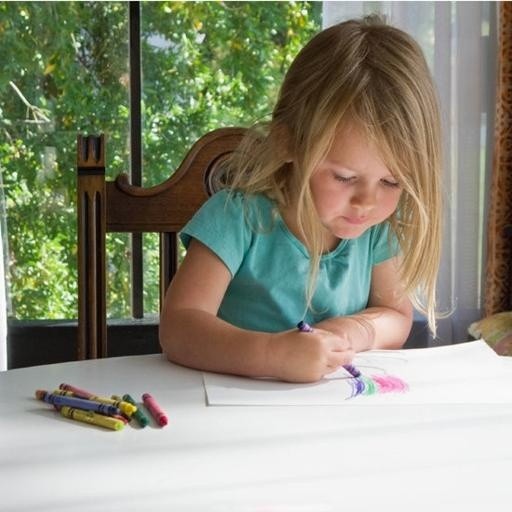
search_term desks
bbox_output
[2,355,509,507]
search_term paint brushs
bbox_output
[36,384,167,430]
[297,321,361,378]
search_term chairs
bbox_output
[75,127,271,360]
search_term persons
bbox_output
[158,13,456,383]
